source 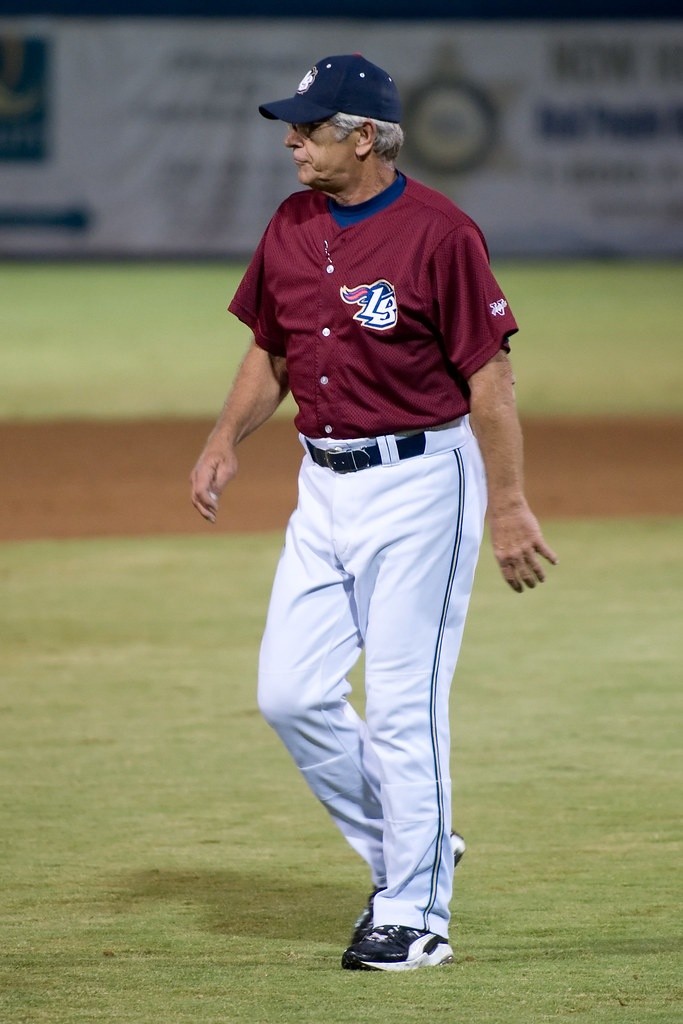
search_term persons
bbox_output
[187,50,563,975]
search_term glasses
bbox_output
[286,121,340,140]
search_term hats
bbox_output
[258,52,403,124]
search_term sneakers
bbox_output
[342,925,455,971]
[351,829,467,945]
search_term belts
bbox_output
[304,431,426,473]
